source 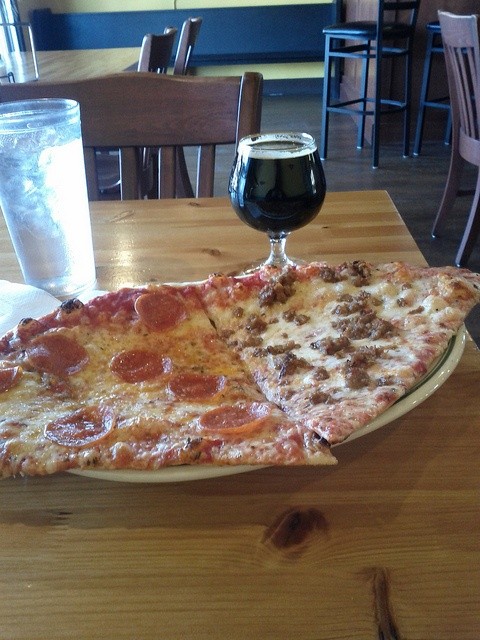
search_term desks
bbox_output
[1,46,142,83]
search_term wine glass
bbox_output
[228,132,327,276]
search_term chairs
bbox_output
[1,71,264,200]
[97,25,178,199]
[144,15,203,198]
[430,9,480,268]
[414,22,472,156]
[320,0,421,170]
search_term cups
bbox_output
[0,99,98,296]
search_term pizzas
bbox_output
[2,261,480,482]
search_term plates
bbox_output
[64,281,466,484]
